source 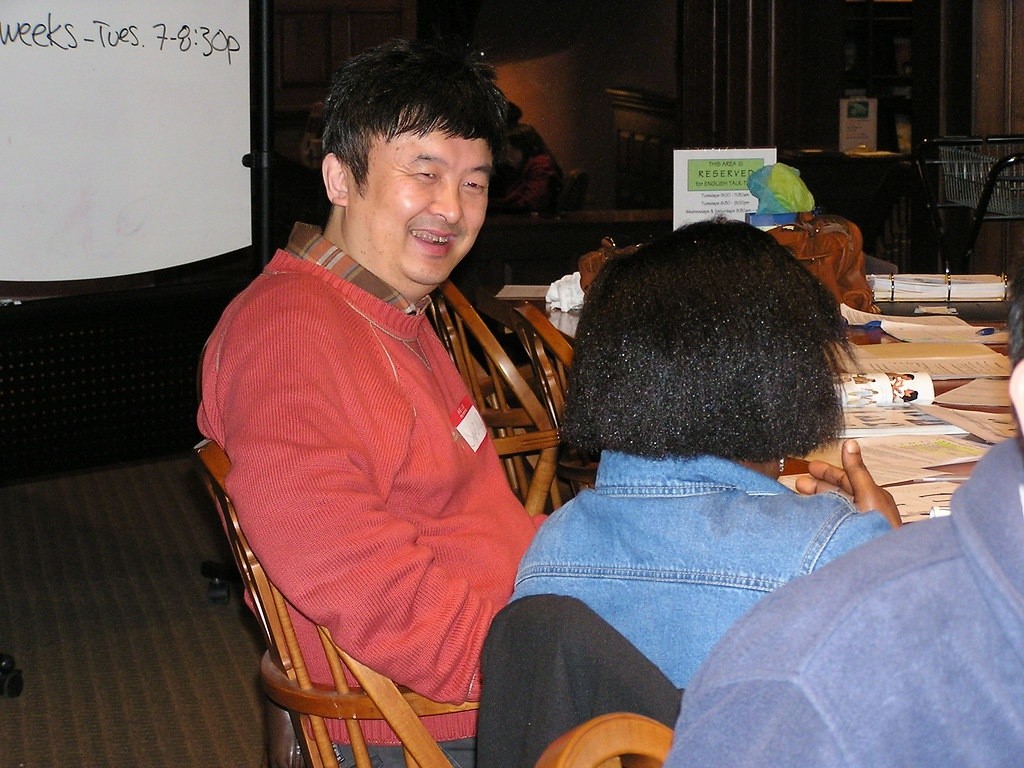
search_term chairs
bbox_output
[533,712,674,768]
[428,280,600,510]
[195,436,482,768]
[512,306,576,429]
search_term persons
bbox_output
[661,270,1024,768]
[892,384,918,402]
[510,223,902,694]
[487,104,562,214]
[885,372,914,383]
[196,37,548,768]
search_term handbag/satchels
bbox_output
[576,236,648,296]
[762,209,874,319]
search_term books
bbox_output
[802,269,1012,439]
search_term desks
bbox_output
[777,319,1020,527]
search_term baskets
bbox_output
[938,142,1024,216]
